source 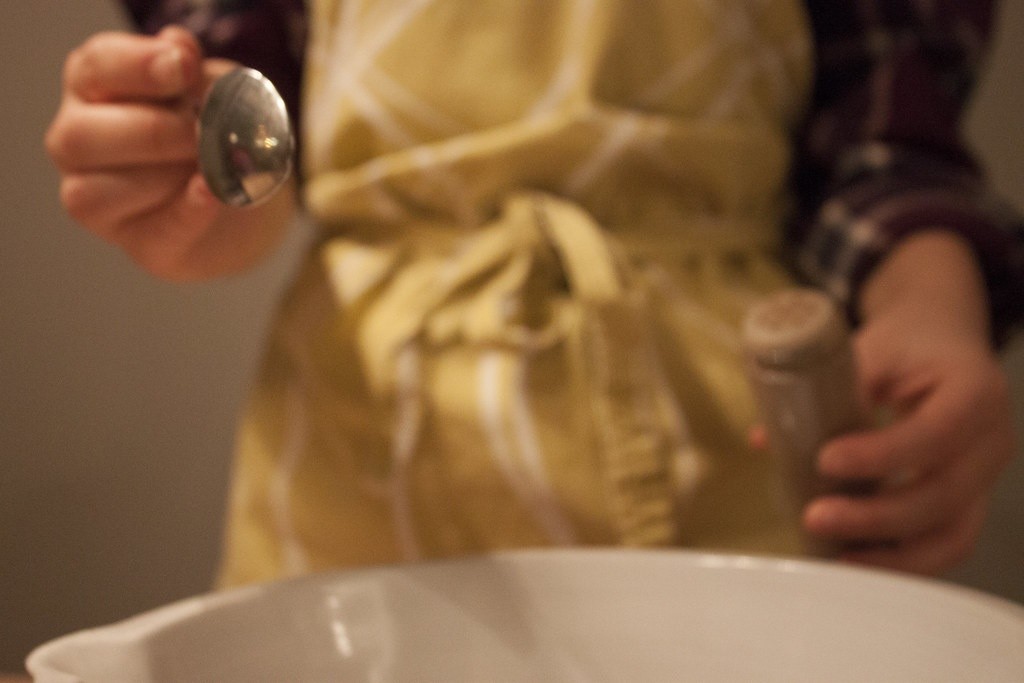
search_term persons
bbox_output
[44,0,1024,593]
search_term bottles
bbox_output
[742,288,895,562]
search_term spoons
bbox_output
[151,66,295,211]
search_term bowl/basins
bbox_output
[22,545,1024,682]
